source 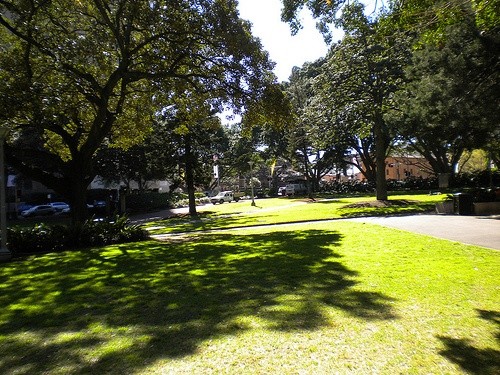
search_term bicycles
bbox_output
[336,179,376,198]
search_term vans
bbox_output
[277,187,286,195]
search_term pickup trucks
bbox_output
[211,191,246,205]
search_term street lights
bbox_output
[247,160,256,206]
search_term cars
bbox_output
[15,201,105,217]
